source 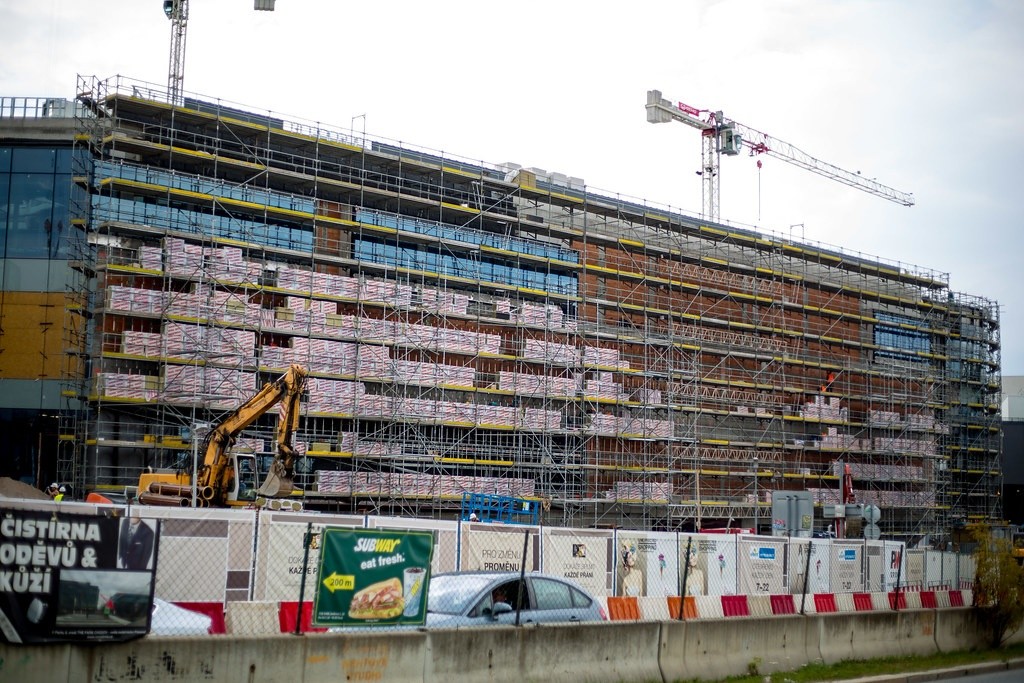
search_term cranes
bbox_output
[166,0,190,108]
[643,89,916,224]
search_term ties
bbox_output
[126,526,134,552]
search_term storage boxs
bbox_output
[91,90,949,506]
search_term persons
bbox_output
[47,483,66,502]
[818,369,835,396]
[621,541,643,597]
[682,543,705,597]
[481,586,510,616]
[795,543,806,593]
[119,517,154,570]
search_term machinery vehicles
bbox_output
[138,363,309,507]
[1011,532,1024,565]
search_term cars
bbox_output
[328,571,609,633]
[150,596,216,637]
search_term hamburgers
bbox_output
[349,578,405,619]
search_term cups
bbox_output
[403,567,427,617]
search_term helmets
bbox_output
[50,483,58,488]
[469,513,476,519]
[59,487,66,492]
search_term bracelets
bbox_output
[48,488,50,489]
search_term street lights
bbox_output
[869,476,875,539]
[752,456,760,534]
